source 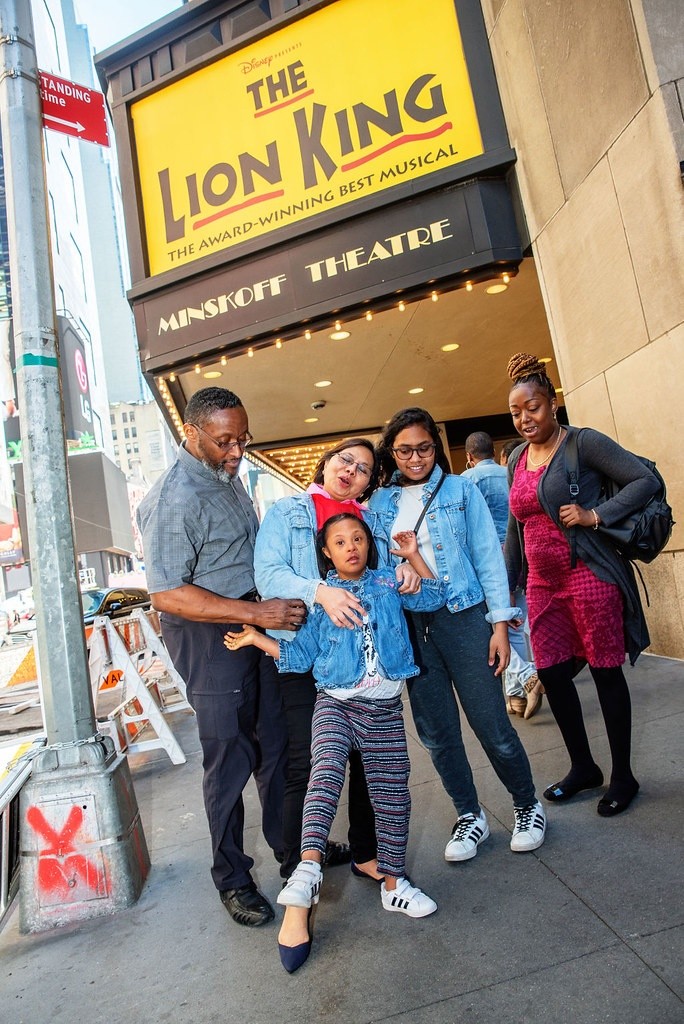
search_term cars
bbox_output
[7,585,152,644]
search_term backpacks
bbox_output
[566,427,677,565]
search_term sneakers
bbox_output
[511,800,547,851]
[444,808,489,861]
[380,876,438,917]
[276,860,324,908]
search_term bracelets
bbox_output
[591,509,598,530]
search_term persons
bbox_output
[357,408,547,861]
[504,352,660,818]
[459,432,546,721]
[136,387,349,927]
[252,439,422,974]
[224,512,446,918]
[500,440,526,467]
[12,610,19,625]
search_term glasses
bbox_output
[189,422,254,452]
[391,442,438,460]
[333,452,373,477]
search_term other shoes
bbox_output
[350,860,415,886]
[597,778,640,817]
[278,904,313,975]
[524,672,546,720]
[543,764,604,802]
[506,696,527,717]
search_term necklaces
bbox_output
[529,426,561,466]
[405,485,422,501]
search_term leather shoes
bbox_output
[322,838,351,867]
[219,880,275,927]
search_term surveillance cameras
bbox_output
[310,401,326,410]
